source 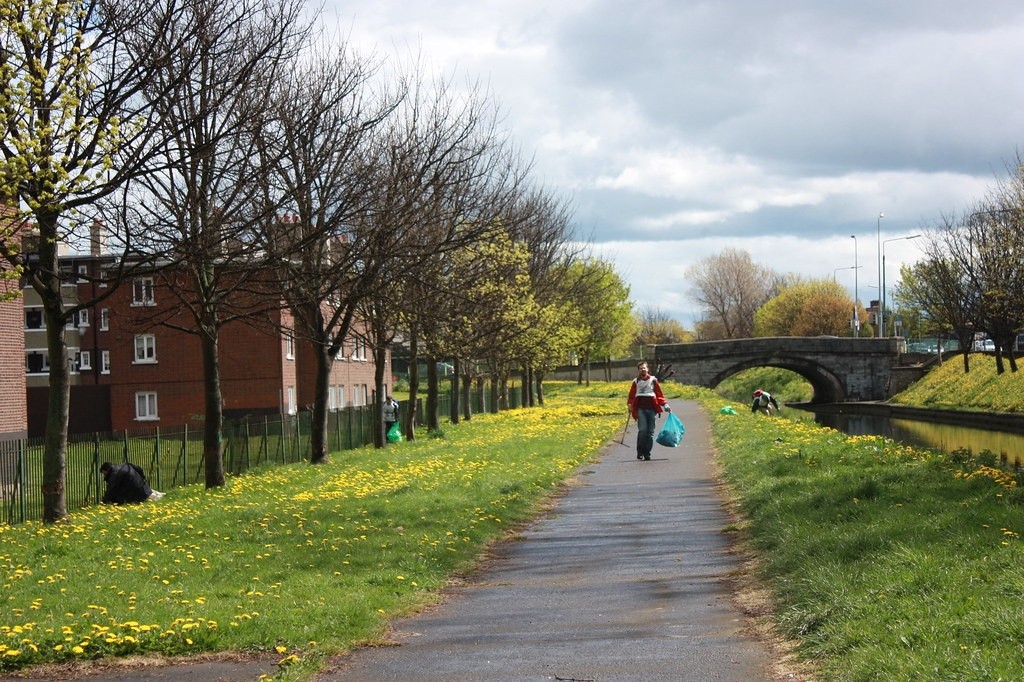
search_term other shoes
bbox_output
[645,456,650,460]
[637,455,643,459]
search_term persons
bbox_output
[752,392,780,416]
[100,462,153,506]
[627,361,671,461]
[383,396,399,442]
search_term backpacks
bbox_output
[752,390,763,399]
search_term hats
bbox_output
[763,393,768,400]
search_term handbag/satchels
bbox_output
[656,412,686,447]
[387,421,401,442]
